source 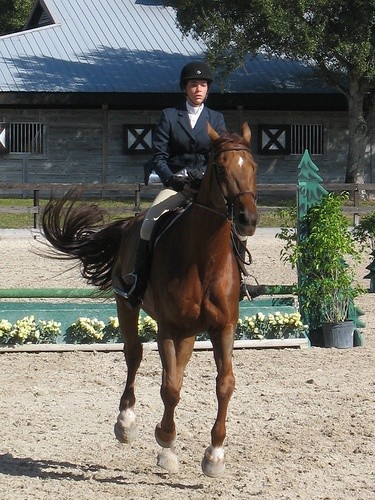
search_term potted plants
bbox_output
[353,207,375,293]
[276,189,362,350]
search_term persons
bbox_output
[112,62,266,310]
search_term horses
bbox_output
[27,121,257,478]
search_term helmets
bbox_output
[181,62,212,87]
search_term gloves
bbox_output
[188,171,204,188]
[168,174,185,191]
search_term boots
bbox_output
[113,237,154,309]
[236,240,267,301]
